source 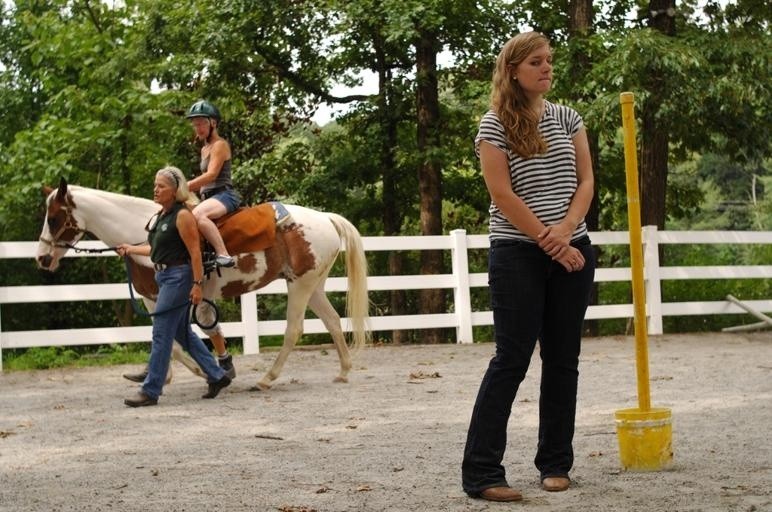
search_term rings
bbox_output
[572,259,576,265]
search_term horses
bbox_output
[36,176,369,392]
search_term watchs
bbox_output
[192,280,204,285]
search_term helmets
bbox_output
[186,101,221,119]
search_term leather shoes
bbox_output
[218,355,236,379]
[125,390,158,407]
[544,477,571,492]
[202,375,231,400]
[482,486,523,501]
[122,365,150,381]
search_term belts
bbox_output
[154,256,189,271]
[201,185,233,199]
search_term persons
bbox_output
[123,302,236,382]
[185,99,242,268]
[121,166,237,407]
[461,30,596,503]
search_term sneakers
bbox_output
[204,256,238,272]
